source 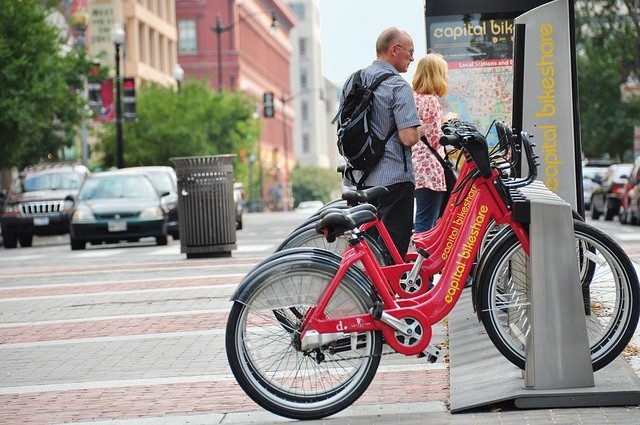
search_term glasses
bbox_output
[392,41,415,56]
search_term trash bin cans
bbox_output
[168,153,238,260]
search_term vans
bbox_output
[581,158,616,211]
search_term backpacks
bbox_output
[330,69,397,189]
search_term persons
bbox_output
[411,52,474,291]
[337,27,426,302]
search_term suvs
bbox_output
[1,161,91,248]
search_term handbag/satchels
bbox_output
[444,159,457,193]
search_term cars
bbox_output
[121,164,181,238]
[65,173,170,248]
[297,200,323,208]
[619,156,639,223]
[590,162,634,221]
